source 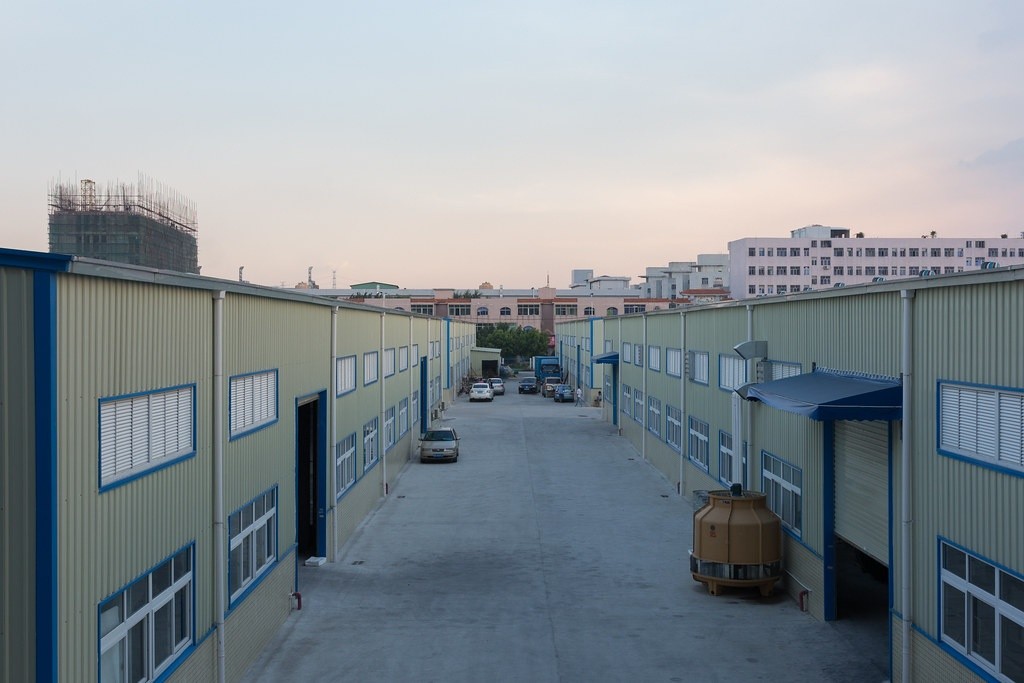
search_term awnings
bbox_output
[745,371,902,421]
[590,351,618,365]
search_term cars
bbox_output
[518,377,541,394]
[487,377,506,395]
[469,382,495,402]
[540,376,563,398]
[554,384,575,402]
[418,426,461,462]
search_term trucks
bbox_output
[535,356,563,384]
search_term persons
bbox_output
[575,387,583,407]
[558,383,565,402]
[594,391,602,407]
